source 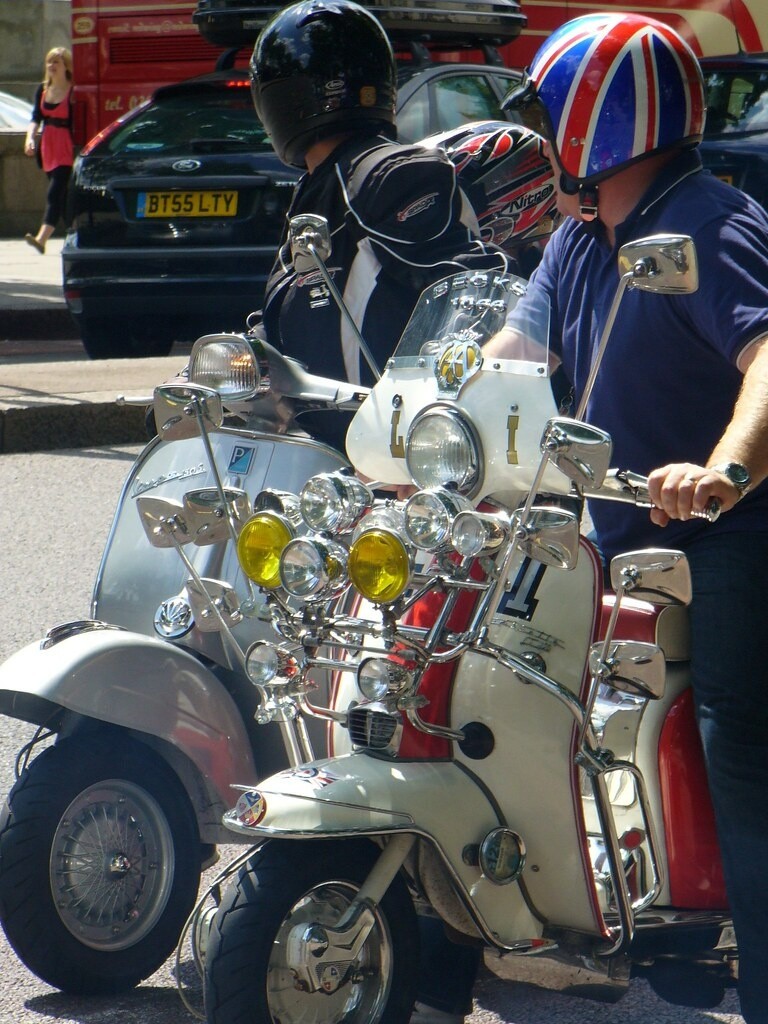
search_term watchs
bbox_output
[713,461,752,503]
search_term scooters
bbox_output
[129,211,767,1024]
[1,328,354,998]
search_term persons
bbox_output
[24,46,72,256]
[143,0,768,1024]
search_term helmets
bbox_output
[428,122,563,250]
[249,1,398,166]
[499,13,706,189]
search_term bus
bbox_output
[66,0,768,155]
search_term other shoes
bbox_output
[26,233,46,254]
[409,1002,465,1024]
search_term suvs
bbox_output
[62,0,550,362]
[693,51,768,212]
